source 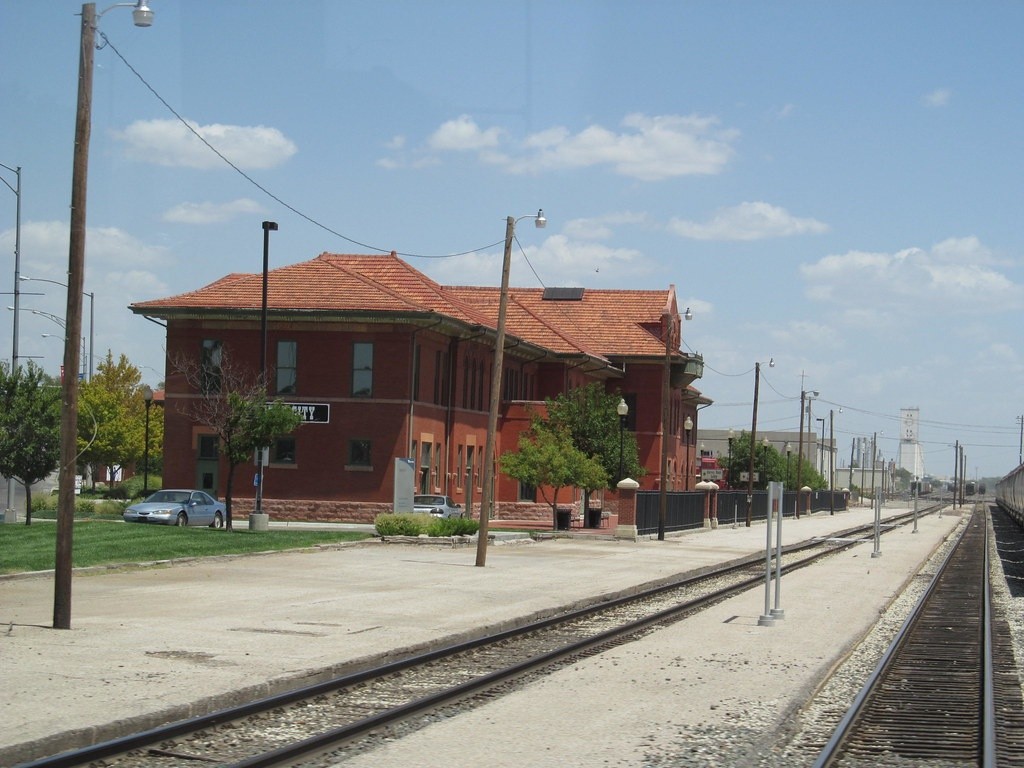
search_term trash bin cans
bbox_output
[557,509,572,530]
[588,507,603,529]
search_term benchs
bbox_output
[570,516,581,528]
[600,513,609,528]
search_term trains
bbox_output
[966,482,978,495]
[910,481,931,495]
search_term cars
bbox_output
[123,488,227,528]
[414,494,463,522]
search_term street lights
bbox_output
[699,441,705,482]
[727,427,735,490]
[616,398,629,481]
[816,418,825,491]
[18,275,95,387]
[761,436,769,490]
[870,431,884,508]
[50,0,153,630]
[795,390,820,520]
[658,308,693,541]
[684,417,693,492]
[8,306,86,387]
[744,358,775,527]
[142,385,153,499]
[786,442,792,490]
[250,219,278,532]
[473,209,546,567]
[829,408,843,516]
